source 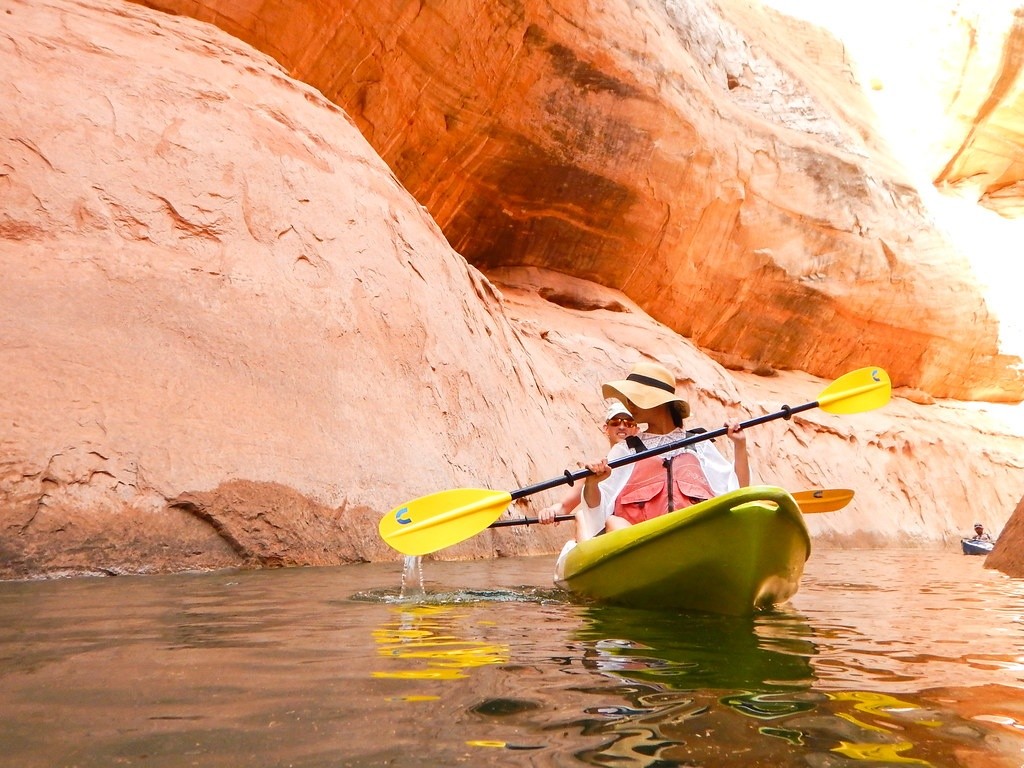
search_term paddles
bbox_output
[489,487,855,528]
[378,363,894,557]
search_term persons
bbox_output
[539,402,640,543]
[581,364,751,542]
[972,523,992,541]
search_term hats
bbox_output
[601,363,690,419]
[606,403,635,422]
[974,523,984,530]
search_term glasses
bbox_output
[607,418,638,428]
[626,397,634,406]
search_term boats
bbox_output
[552,484,813,619]
[960,537,994,556]
